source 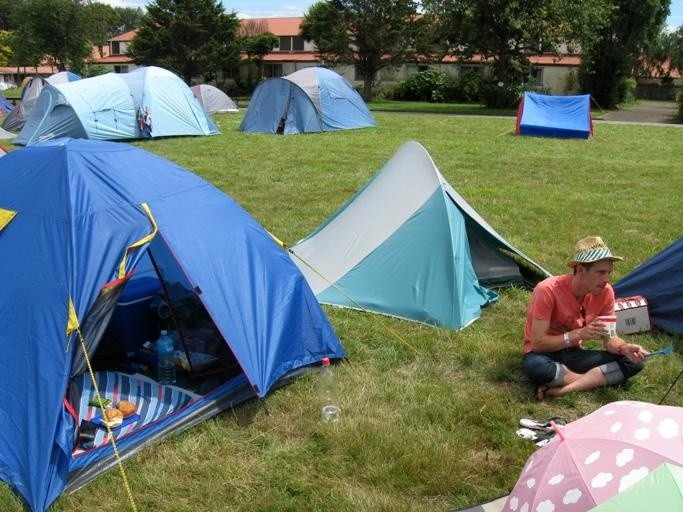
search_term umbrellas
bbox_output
[497,397,683,511]
[583,461,683,512]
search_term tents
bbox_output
[0,136,346,510]
[610,238,682,339]
[19,70,82,103]
[8,66,221,148]
[513,90,594,141]
[0,97,38,131]
[283,141,556,331]
[232,66,377,135]
[189,83,239,119]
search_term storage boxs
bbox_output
[113,277,168,347]
[615,295,652,334]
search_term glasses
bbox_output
[581,306,586,328]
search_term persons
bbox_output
[523,237,651,402]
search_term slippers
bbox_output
[537,386,551,401]
[520,416,566,431]
[517,428,556,448]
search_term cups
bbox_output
[594,314,618,341]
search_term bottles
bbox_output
[316,357,342,424]
[155,328,178,386]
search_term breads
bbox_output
[115,400,137,419]
[102,408,124,429]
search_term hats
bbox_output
[566,237,624,268]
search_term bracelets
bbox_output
[562,332,571,347]
[618,343,625,356]
